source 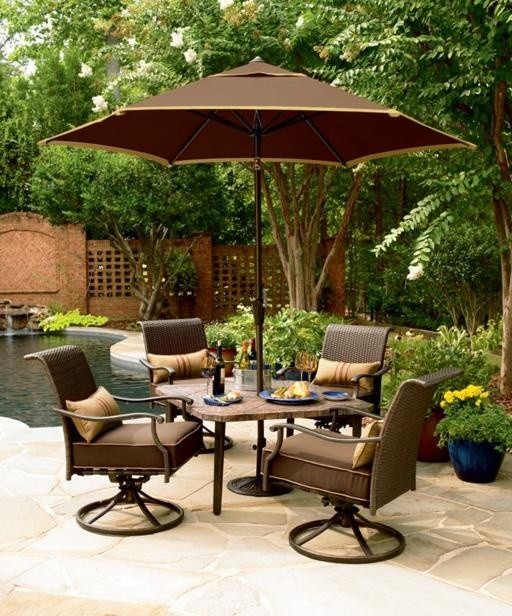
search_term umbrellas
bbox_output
[37,54,476,476]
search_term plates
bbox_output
[260,389,318,405]
[203,392,243,407]
[321,390,348,401]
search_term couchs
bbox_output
[287,324,392,439]
[259,369,461,564]
[139,320,234,454]
[25,345,200,535]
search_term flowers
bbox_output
[436,385,512,450]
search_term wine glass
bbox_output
[303,352,316,387]
[201,357,215,396]
[295,352,307,381]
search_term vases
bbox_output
[447,438,504,483]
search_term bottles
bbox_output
[212,339,225,395]
[239,340,250,368]
[249,338,257,369]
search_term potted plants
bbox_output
[409,328,487,461]
